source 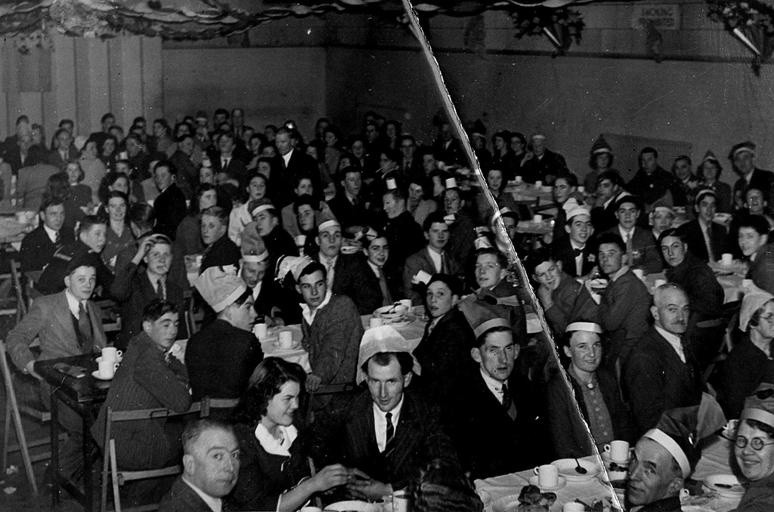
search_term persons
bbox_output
[247,197,299,258]
[148,161,186,240]
[0,112,170,160]
[100,190,137,276]
[459,247,527,338]
[222,355,349,512]
[37,215,114,302]
[719,280,774,411]
[5,258,113,498]
[626,408,699,512]
[312,200,357,297]
[740,184,774,234]
[547,199,599,279]
[364,107,436,190]
[595,233,652,375]
[19,195,68,273]
[108,231,183,351]
[436,122,581,210]
[453,303,542,478]
[229,173,266,247]
[304,117,364,166]
[597,192,662,276]
[671,154,699,205]
[328,166,374,231]
[692,150,732,212]
[548,321,623,459]
[404,179,436,228]
[158,418,241,512]
[734,393,774,512]
[239,222,272,314]
[326,324,447,501]
[192,206,241,313]
[349,230,391,316]
[590,170,634,229]
[647,201,677,241]
[657,228,724,364]
[413,273,479,405]
[0,160,148,214]
[382,189,426,266]
[175,183,218,262]
[585,133,619,189]
[115,202,158,276]
[293,193,320,259]
[523,247,597,334]
[395,211,465,306]
[724,215,774,295]
[489,206,524,258]
[270,126,320,197]
[628,146,670,204]
[678,188,727,267]
[282,177,316,233]
[90,297,190,509]
[293,261,365,411]
[727,141,774,214]
[620,282,693,428]
[185,263,264,400]
[170,106,275,178]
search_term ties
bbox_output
[78,303,94,335]
[427,321,436,337]
[327,262,333,277]
[681,338,693,360]
[706,221,718,259]
[441,254,446,274]
[223,160,228,168]
[378,267,391,304]
[55,233,61,242]
[64,149,67,160]
[385,413,394,445]
[625,234,633,258]
[156,279,167,301]
[502,385,516,419]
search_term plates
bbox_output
[681,505,714,512]
[373,304,409,321]
[324,500,371,512]
[96,356,122,363]
[341,245,358,254]
[92,370,116,380]
[591,278,608,288]
[721,430,739,441]
[601,453,629,469]
[704,474,746,495]
[491,494,520,512]
[392,322,408,327]
[551,458,601,482]
[273,342,299,350]
[529,476,568,492]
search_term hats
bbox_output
[701,149,719,164]
[460,293,510,337]
[247,198,273,218]
[694,183,716,200]
[739,382,774,426]
[565,282,602,331]
[192,266,246,313]
[613,186,633,202]
[240,222,268,263]
[147,222,173,247]
[644,401,701,477]
[314,200,340,229]
[738,277,774,331]
[651,196,675,216]
[489,199,510,225]
[593,134,610,153]
[278,255,314,286]
[355,325,423,383]
[563,198,590,219]
[730,141,757,155]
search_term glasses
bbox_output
[734,433,774,450]
[475,262,498,271]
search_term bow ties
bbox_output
[572,248,584,255]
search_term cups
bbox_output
[370,317,387,328]
[393,489,407,512]
[651,279,667,292]
[633,269,644,280]
[102,347,123,363]
[99,361,120,378]
[563,502,584,512]
[401,300,412,312]
[295,234,307,246]
[679,488,689,503]
[515,176,522,182]
[603,440,630,462]
[536,180,541,187]
[533,214,542,223]
[534,464,558,488]
[278,331,292,348]
[722,419,739,437]
[578,186,585,193]
[252,324,267,339]
[719,253,733,267]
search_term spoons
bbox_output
[573,457,589,474]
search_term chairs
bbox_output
[303,384,355,425]
[0,341,70,498]
[210,396,242,408]
[98,396,212,511]
[10,257,28,324]
